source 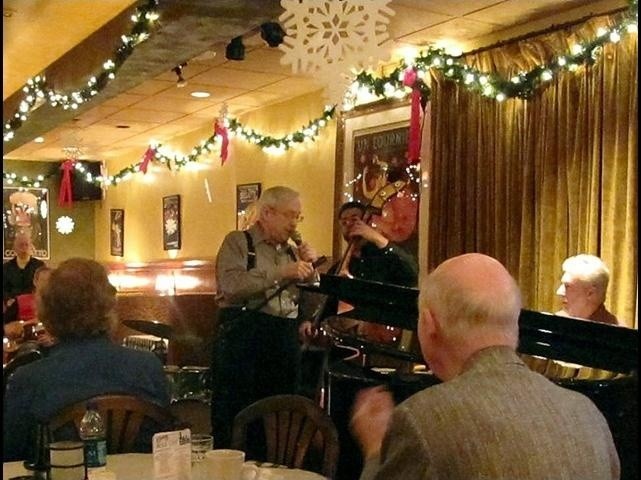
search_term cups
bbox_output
[202,448,246,479]
[190,433,214,474]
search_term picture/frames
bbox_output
[236,184,261,231]
[162,194,181,250]
[110,208,124,257]
[333,92,420,274]
[3,187,50,264]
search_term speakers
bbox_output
[69,160,103,200]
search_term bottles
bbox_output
[79,399,106,480]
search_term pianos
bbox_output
[296,274,640,479]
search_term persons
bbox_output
[348,251,621,480]
[3,232,48,310]
[3,267,60,348]
[1,255,176,459]
[209,186,316,466]
[538,254,629,327]
[299,202,417,348]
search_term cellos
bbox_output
[297,177,410,393]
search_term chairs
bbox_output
[3,349,41,383]
[48,395,174,454]
[232,393,341,478]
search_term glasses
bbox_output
[270,208,305,225]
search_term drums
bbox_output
[164,365,212,406]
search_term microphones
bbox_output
[289,231,302,246]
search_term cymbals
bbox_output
[122,319,202,345]
